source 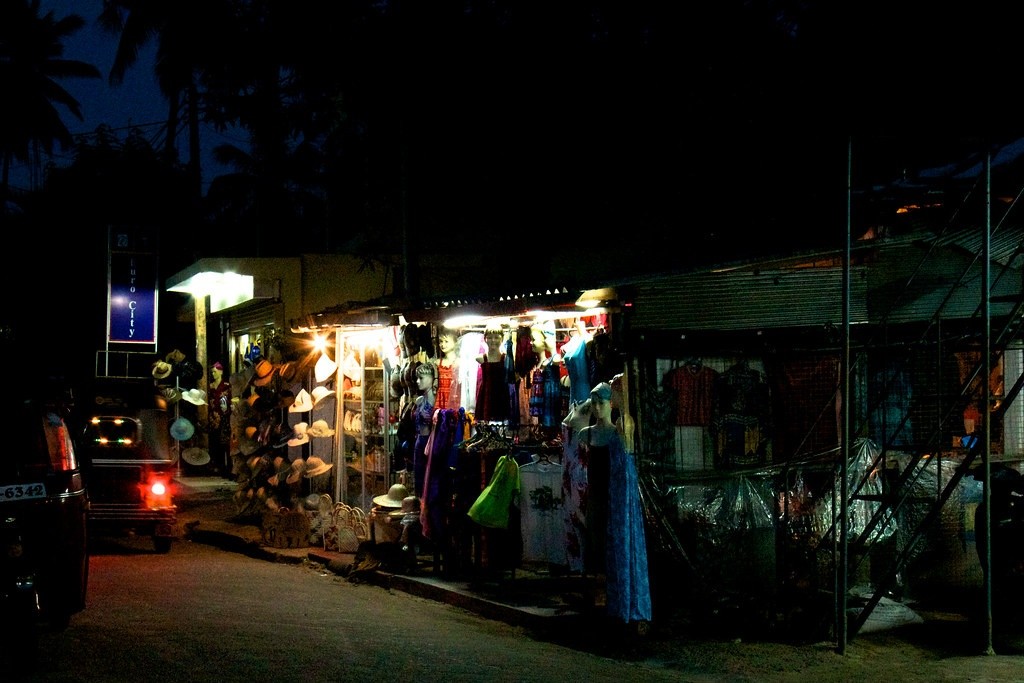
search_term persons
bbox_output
[434,325,465,416]
[476,333,518,422]
[526,319,593,435]
[562,378,636,642]
[406,364,444,573]
[208,362,233,472]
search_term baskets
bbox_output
[260,494,368,553]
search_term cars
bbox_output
[0,412,94,608]
[65,378,182,553]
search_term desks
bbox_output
[367,512,441,577]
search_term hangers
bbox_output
[687,357,702,369]
[736,352,753,372]
[454,423,565,474]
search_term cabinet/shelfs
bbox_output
[293,364,334,496]
[346,356,414,504]
[171,352,189,478]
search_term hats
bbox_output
[390,322,451,474]
[229,346,337,520]
[152,350,210,465]
[590,382,612,400]
[609,373,624,391]
[371,483,424,527]
